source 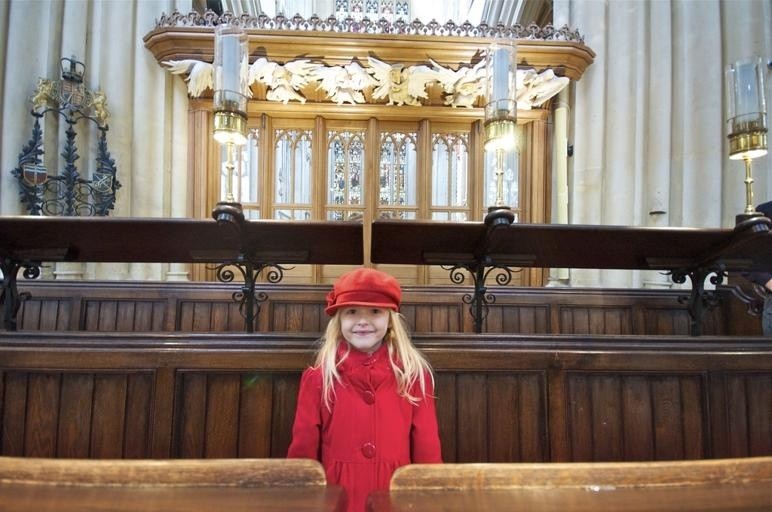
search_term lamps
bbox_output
[484,47,516,223]
[723,58,769,229]
[213,34,249,221]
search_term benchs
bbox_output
[0,456,326,512]
[388,456,772,512]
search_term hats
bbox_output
[324,268,401,316]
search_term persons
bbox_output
[288,268,443,512]
